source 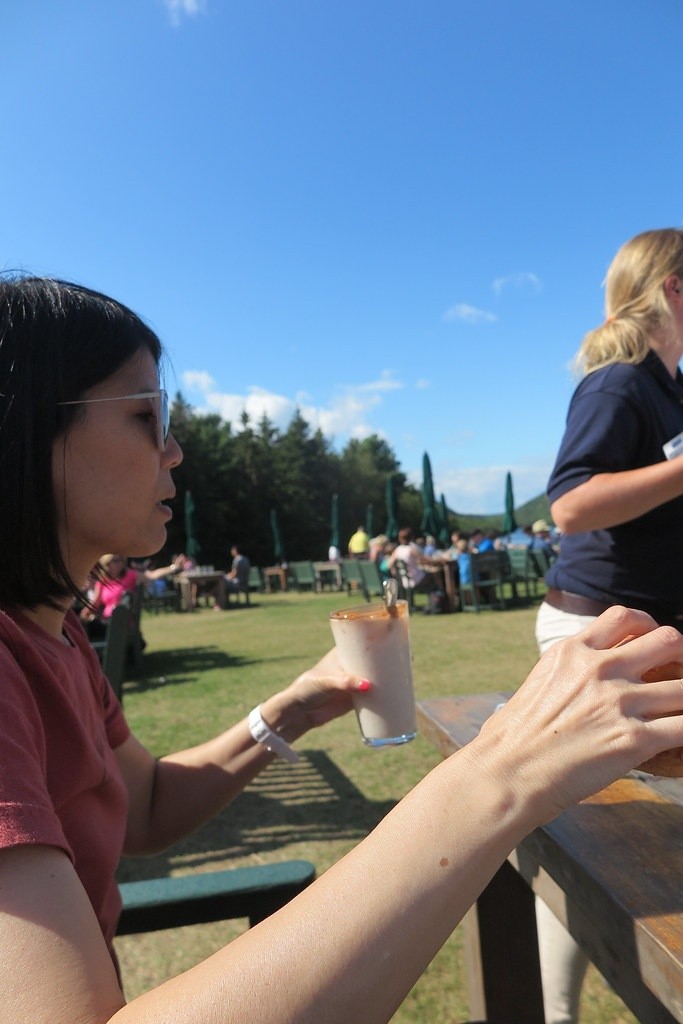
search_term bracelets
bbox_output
[248,703,300,764]
[170,564,175,572]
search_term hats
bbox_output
[531,519,551,533]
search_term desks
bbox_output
[417,697,683,1024]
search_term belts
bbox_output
[543,586,672,625]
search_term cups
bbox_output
[328,598,420,748]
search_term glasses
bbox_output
[60,389,170,452]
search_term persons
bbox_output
[0,278,683,1024]
[213,545,248,609]
[147,555,197,612]
[368,520,557,614]
[536,230,683,654]
[347,526,369,560]
[81,554,185,637]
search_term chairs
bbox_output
[111,858,319,931]
[91,545,559,703]
